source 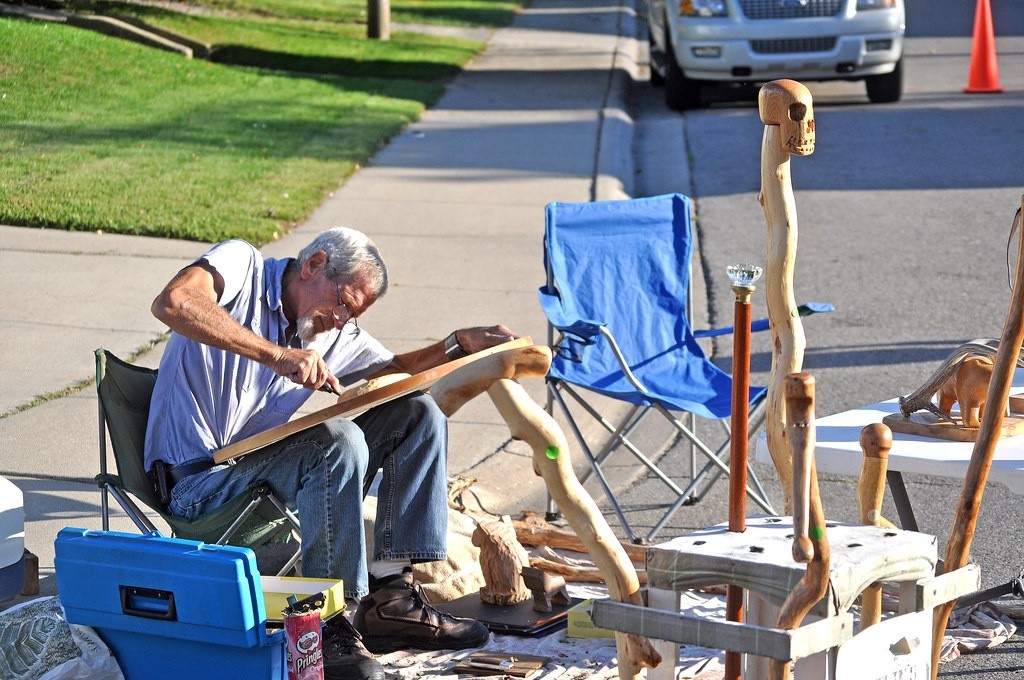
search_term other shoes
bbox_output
[352,567,490,656]
[321,613,385,680]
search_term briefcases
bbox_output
[54,527,327,680]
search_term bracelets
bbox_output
[443,329,470,360]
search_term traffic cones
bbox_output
[962,0,1008,94]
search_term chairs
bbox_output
[541,195,836,543]
[93,348,377,578]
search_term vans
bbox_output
[643,1,907,106]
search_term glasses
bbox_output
[328,257,361,341]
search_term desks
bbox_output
[753,387,1024,613]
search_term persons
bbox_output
[144,224,522,680]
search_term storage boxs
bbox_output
[260,576,344,620]
[53,525,346,680]
